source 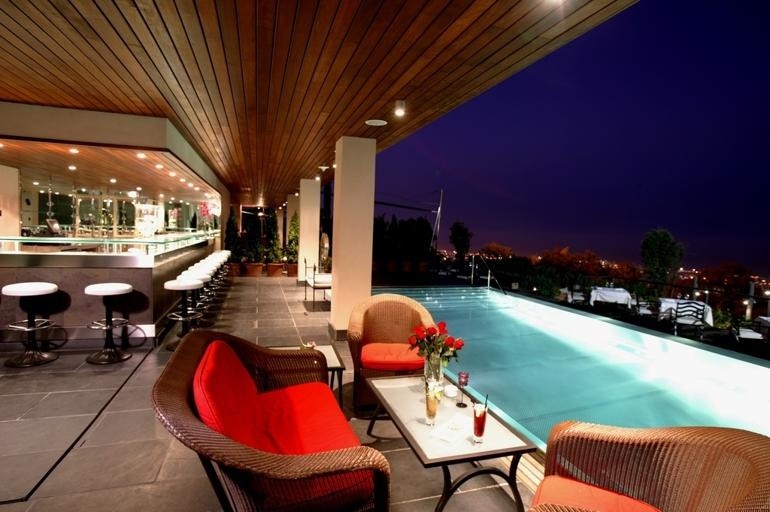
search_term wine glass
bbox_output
[455,372,469,408]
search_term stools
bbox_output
[1,282,133,368]
[164,249,233,352]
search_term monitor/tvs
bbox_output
[46,219,61,233]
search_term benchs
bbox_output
[305,257,332,312]
[150,330,390,512]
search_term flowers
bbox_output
[406,321,464,381]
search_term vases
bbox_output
[423,352,444,417]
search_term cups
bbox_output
[471,404,488,444]
[443,384,458,397]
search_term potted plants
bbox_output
[225,206,300,277]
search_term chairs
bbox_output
[561,268,764,341]
[528,421,770,512]
[346,293,440,416]
